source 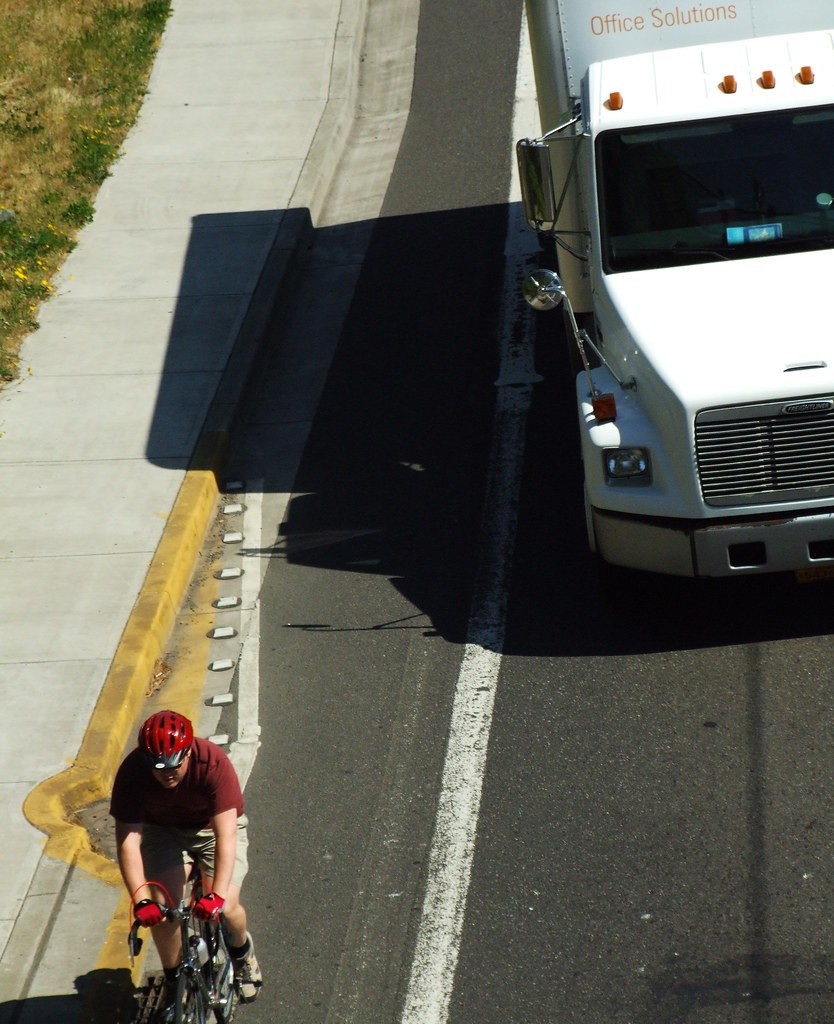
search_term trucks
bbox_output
[516,0,834,583]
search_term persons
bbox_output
[109,709,263,1023]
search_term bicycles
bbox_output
[126,847,242,1023]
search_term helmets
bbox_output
[139,710,193,769]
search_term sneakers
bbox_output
[179,989,195,1023]
[224,931,263,1004]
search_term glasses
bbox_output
[151,756,185,770]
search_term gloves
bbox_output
[192,891,224,922]
[132,898,165,928]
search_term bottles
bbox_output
[197,939,210,979]
